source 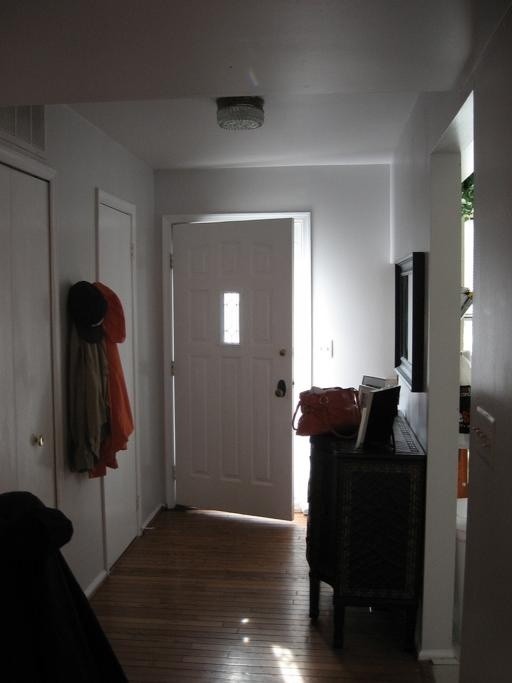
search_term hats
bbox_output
[68,281,108,344]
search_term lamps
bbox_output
[215,96,266,132]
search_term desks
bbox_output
[305,409,424,651]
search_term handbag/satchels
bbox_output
[292,386,362,440]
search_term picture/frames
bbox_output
[393,251,424,393]
